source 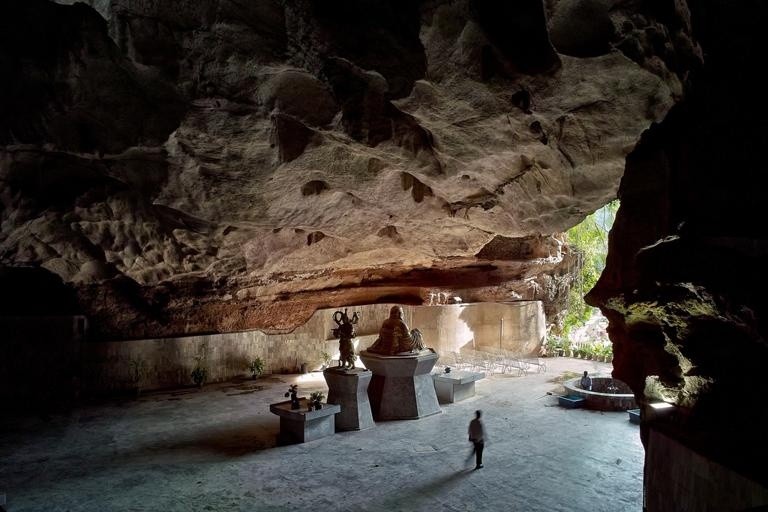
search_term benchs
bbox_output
[443,350,546,376]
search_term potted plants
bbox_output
[285,384,326,411]
[249,356,263,380]
[320,351,331,370]
[192,367,207,388]
[546,337,613,363]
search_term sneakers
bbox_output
[477,465,483,468]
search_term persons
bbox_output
[330,308,360,369]
[464,409,488,469]
[581,371,592,390]
[367,306,427,356]
